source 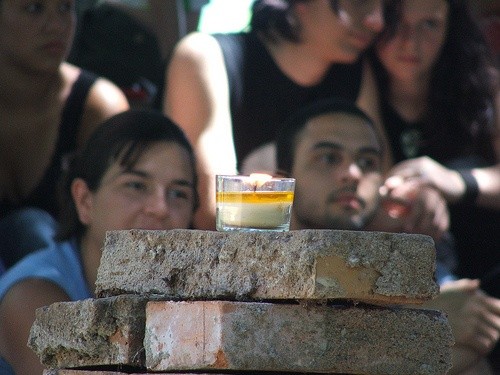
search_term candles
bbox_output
[215,172,293,231]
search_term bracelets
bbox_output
[453,167,479,211]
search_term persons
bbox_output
[276,97,500,374]
[357,0,500,257]
[0,109,199,375]
[0,0,450,242]
